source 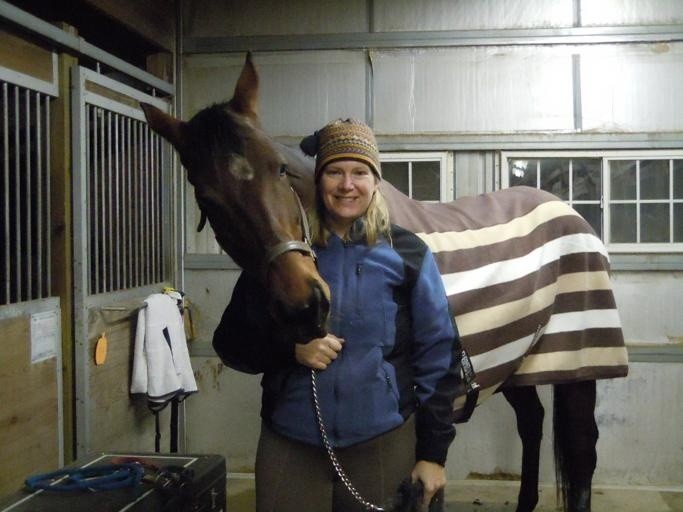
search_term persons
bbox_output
[211,118,463,512]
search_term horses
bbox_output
[137,46,630,512]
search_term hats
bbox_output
[300,118,381,183]
